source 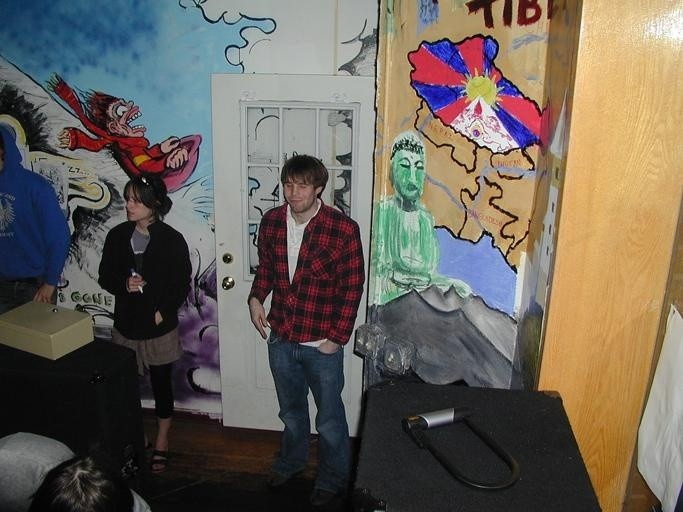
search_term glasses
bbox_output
[140,175,157,193]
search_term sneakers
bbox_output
[269,470,335,505]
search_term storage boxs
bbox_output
[0,299,95,361]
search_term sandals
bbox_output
[150,447,169,473]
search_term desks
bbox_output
[349,380,601,512]
[0,338,147,470]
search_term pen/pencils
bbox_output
[131,268,144,294]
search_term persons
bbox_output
[96,172,191,476]
[247,154,365,509]
[0,122,73,316]
[0,430,150,512]
